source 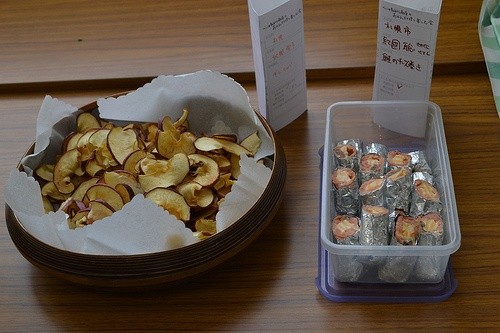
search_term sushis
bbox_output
[332,135,444,283]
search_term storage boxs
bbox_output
[318,100,461,302]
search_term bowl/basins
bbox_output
[4,92,289,290]
